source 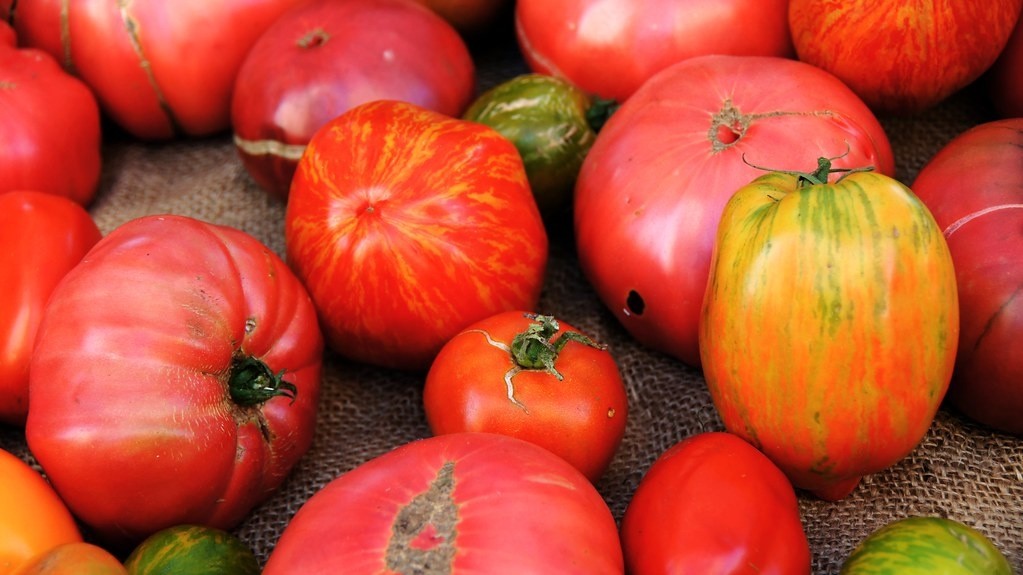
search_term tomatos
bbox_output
[0,0,1023,575]
